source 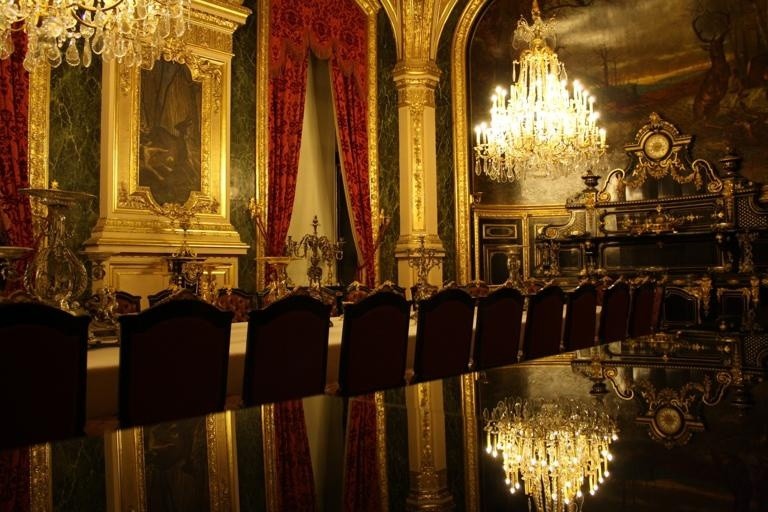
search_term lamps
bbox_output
[485,397,620,512]
[472,1,610,184]
[1,0,192,71]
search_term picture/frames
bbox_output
[0,20,53,293]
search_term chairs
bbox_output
[517,283,564,366]
[109,291,143,312]
[411,282,439,312]
[213,288,264,317]
[2,299,95,445]
[593,282,631,345]
[345,282,368,304]
[625,281,656,339]
[381,280,406,295]
[469,288,526,373]
[116,297,236,431]
[325,291,409,397]
[560,283,598,353]
[229,294,334,408]
[403,288,476,386]
[464,280,489,302]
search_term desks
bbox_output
[1,323,767,511]
